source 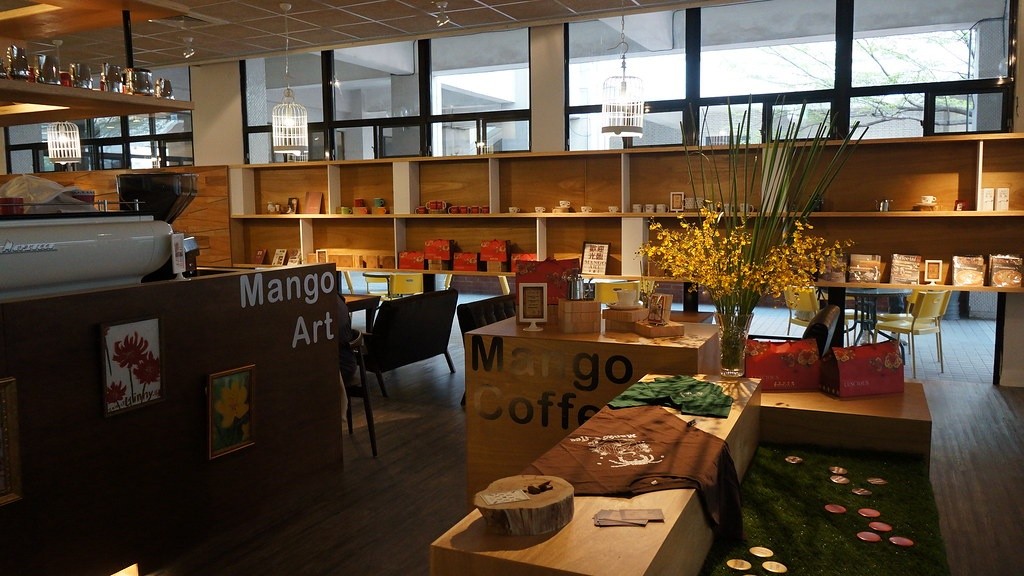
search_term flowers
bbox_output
[632,91,870,371]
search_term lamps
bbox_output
[436,1,449,28]
[47,39,81,165]
[182,37,194,57]
[272,3,308,155]
[601,0,645,137]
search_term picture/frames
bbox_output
[207,364,255,461]
[670,192,684,213]
[0,377,24,507]
[924,260,943,284]
[100,315,167,421]
[315,250,327,264]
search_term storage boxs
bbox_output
[398,239,537,273]
[308,254,395,268]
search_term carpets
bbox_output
[699,442,952,576]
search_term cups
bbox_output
[534,207,546,213]
[341,207,352,215]
[509,207,521,213]
[921,195,937,204]
[740,203,754,212]
[633,204,671,212]
[374,198,384,207]
[724,204,735,213]
[581,206,592,213]
[354,199,364,207]
[609,207,619,213]
[617,290,636,307]
[559,201,570,207]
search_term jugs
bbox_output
[5,45,174,100]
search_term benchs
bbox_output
[344,289,520,460]
[748,306,840,359]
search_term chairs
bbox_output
[784,286,953,379]
[595,280,640,304]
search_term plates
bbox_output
[917,202,937,206]
[606,302,640,309]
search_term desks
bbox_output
[345,294,382,333]
[814,281,912,365]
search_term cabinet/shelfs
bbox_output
[230,131,1024,293]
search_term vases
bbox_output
[714,314,753,376]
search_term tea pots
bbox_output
[875,198,894,211]
[557,269,593,302]
